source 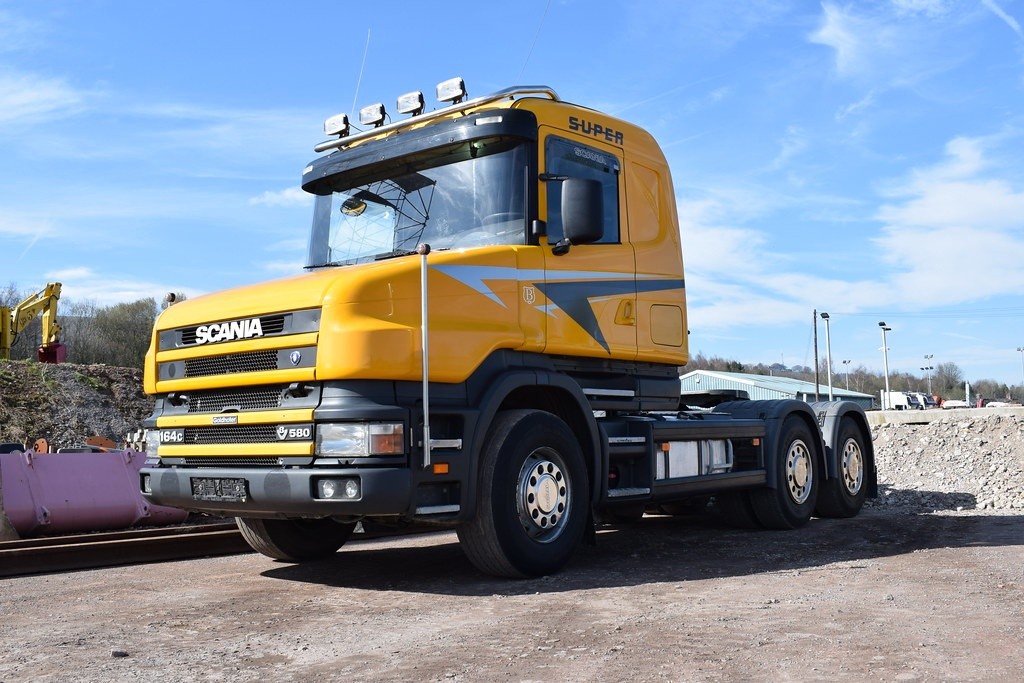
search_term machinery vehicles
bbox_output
[0,282,69,365]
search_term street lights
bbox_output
[920,354,934,397]
[877,321,892,409]
[819,311,834,403]
[841,359,851,392]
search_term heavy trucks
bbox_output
[144,77,891,580]
